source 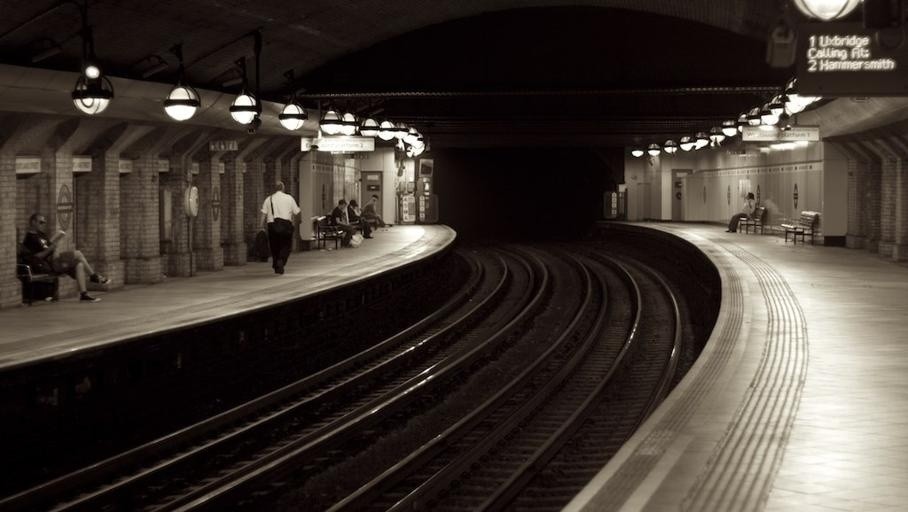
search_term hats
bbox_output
[350,200,358,207]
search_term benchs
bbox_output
[739,206,766,235]
[316,215,343,250]
[780,210,819,245]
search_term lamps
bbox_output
[277,68,427,159]
[71,23,263,126]
[629,89,784,159]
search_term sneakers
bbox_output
[274,260,284,274]
[80,293,100,304]
[89,275,111,285]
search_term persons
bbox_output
[330,198,356,248]
[257,180,303,275]
[724,191,756,234]
[20,210,113,303]
[347,199,374,239]
[361,194,386,228]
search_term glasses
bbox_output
[38,219,45,224]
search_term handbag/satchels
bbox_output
[273,218,294,233]
[349,234,363,247]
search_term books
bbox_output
[49,230,65,243]
